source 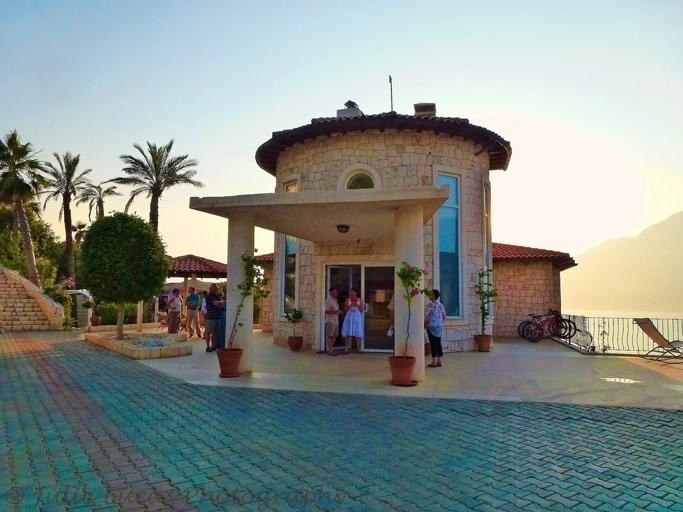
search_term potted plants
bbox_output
[284,308,304,352]
[474,264,498,352]
[215,248,273,378]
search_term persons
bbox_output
[323,286,342,356]
[167,283,226,352]
[387,292,394,356]
[341,287,364,354]
[423,289,446,367]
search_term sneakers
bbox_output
[428,361,441,367]
[206,346,216,352]
[190,334,205,339]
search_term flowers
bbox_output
[395,256,434,360]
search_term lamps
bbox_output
[335,224,349,234]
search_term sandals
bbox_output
[328,350,337,356]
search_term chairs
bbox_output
[155,311,205,332]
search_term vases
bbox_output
[388,355,416,386]
[425,341,431,356]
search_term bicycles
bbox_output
[517,308,578,344]
